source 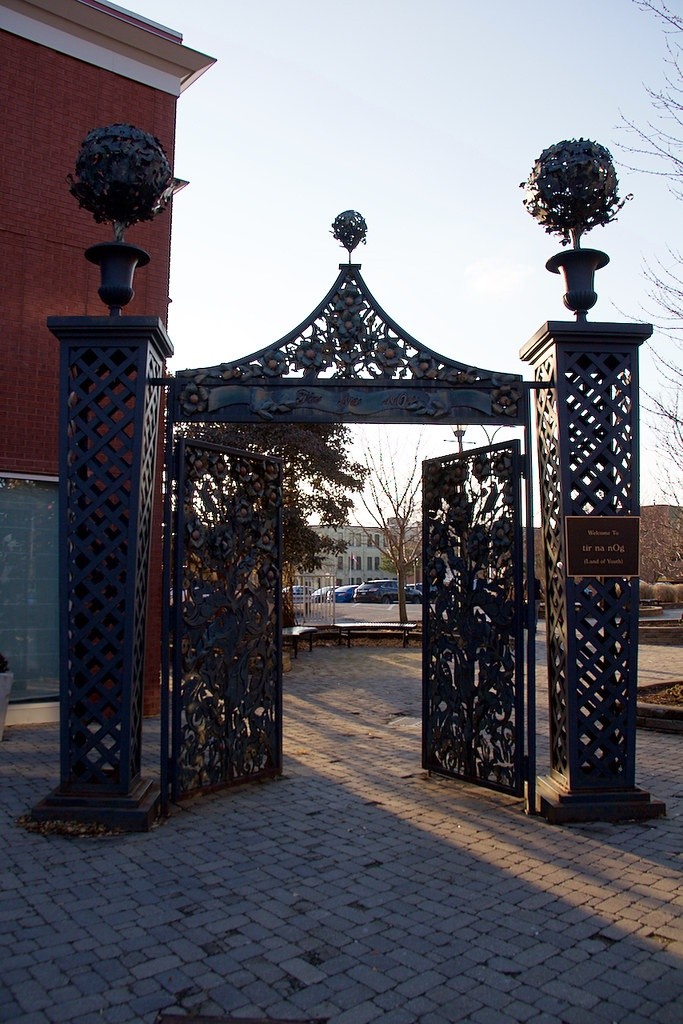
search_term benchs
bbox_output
[333,622,417,647]
[280,627,318,659]
[639,598,663,607]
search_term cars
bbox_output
[309,586,339,601]
[352,579,423,605]
[282,586,316,601]
[330,586,359,602]
